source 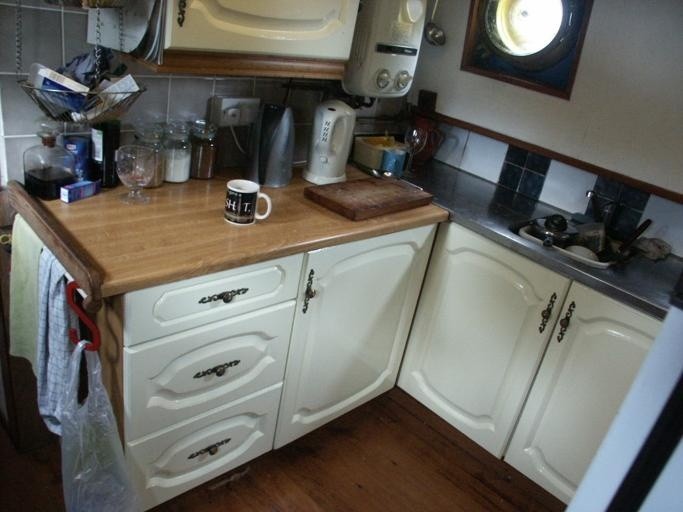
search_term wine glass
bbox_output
[118,145,156,206]
[403,127,428,176]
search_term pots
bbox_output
[530,215,580,248]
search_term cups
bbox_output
[223,179,273,226]
[383,149,404,176]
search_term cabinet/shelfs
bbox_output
[119,0,362,80]
[123,252,304,511]
[397,221,662,506]
[274,224,438,452]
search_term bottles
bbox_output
[191,120,217,178]
[134,124,165,188]
[91,120,120,189]
[162,121,191,182]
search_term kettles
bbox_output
[302,100,356,187]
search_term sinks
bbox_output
[517,214,627,269]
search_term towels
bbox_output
[37,249,81,438]
[7,214,43,377]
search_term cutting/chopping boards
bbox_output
[305,176,433,221]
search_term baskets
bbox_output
[18,79,147,124]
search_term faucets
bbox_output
[586,189,613,216]
